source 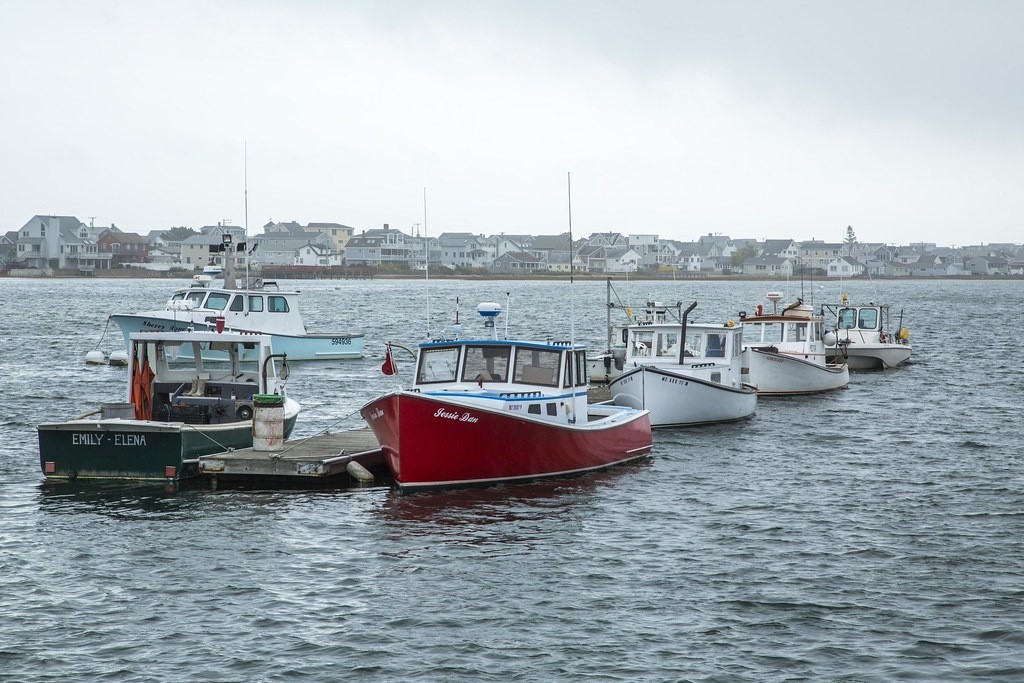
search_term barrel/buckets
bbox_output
[252,404,285,451]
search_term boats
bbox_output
[106,141,365,364]
[360,169,653,496]
[587,345,626,383]
[819,230,912,370]
[37,317,302,482]
[739,252,850,397]
[604,255,758,429]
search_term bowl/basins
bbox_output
[253,395,285,404]
